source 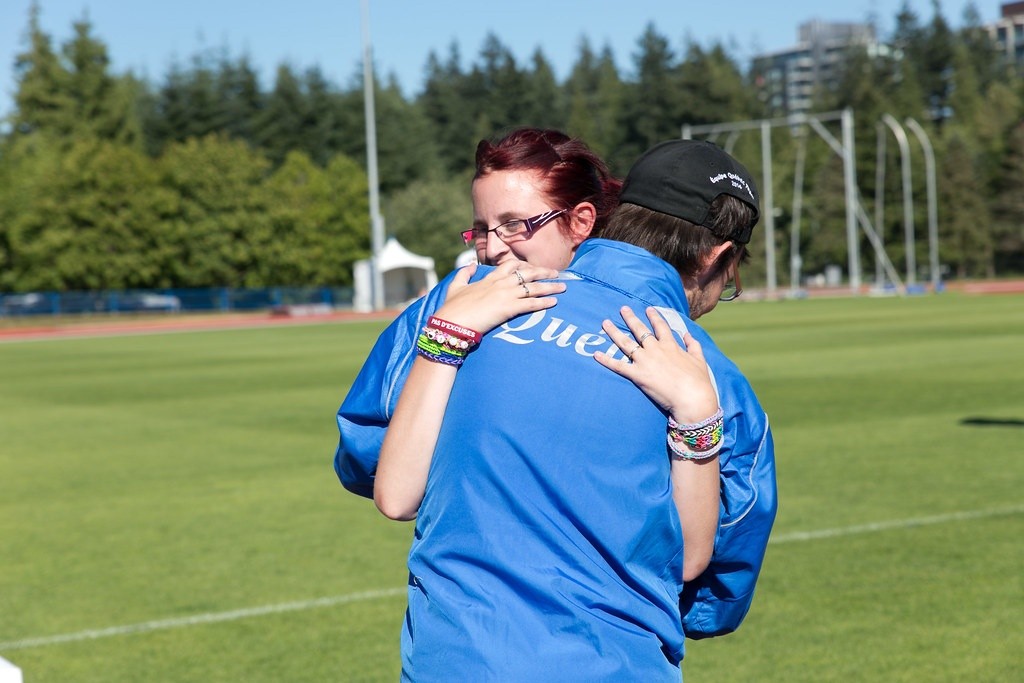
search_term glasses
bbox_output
[459,207,569,249]
[719,253,744,301]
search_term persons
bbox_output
[374,129,724,584]
[333,141,778,682]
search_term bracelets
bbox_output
[416,315,482,365]
[667,408,725,459]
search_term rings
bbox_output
[639,333,655,346]
[511,269,529,298]
[629,346,643,361]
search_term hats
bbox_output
[618,138,762,244]
